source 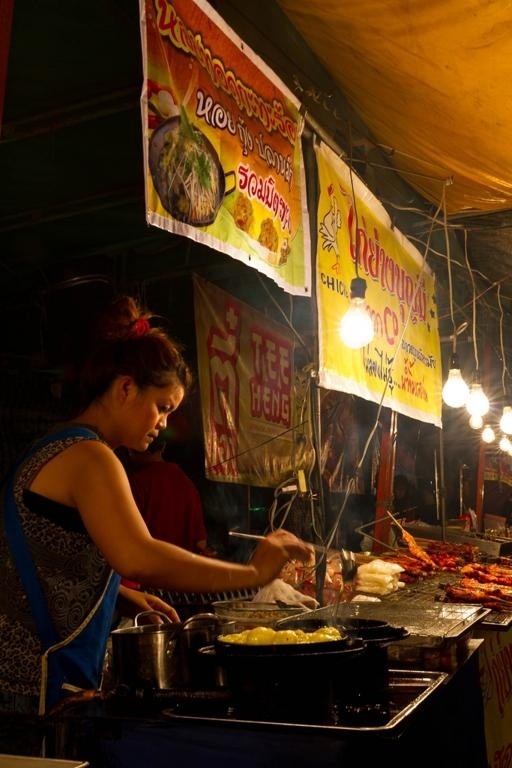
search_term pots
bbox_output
[147,100,236,226]
[276,617,409,716]
[197,632,369,719]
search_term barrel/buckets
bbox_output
[111,609,237,692]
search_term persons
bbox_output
[128,436,209,552]
[393,474,408,500]
[1,297,316,758]
